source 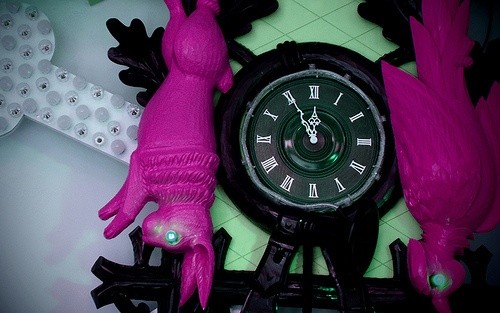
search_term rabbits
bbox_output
[97,0,236,313]
[381,0,499,313]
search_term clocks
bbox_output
[214,41,404,242]
